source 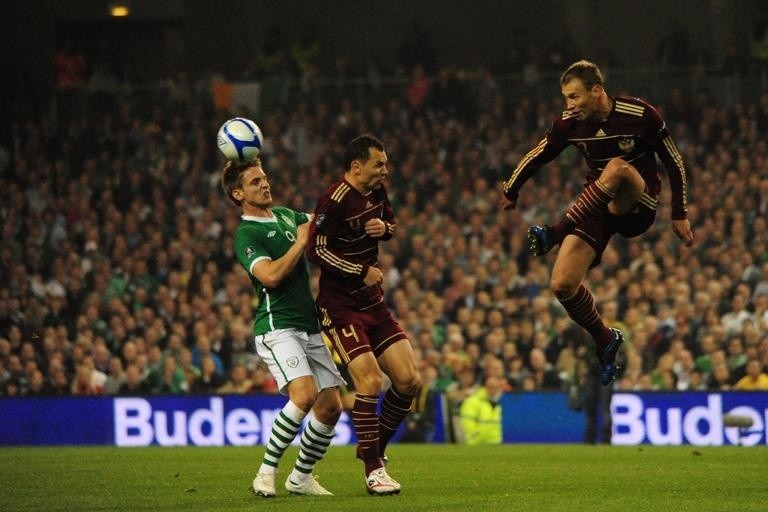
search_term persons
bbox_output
[399,370,438,442]
[219,154,342,499]
[445,368,480,444]
[305,133,424,497]
[459,377,505,444]
[0,22,768,396]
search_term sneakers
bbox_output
[251,471,276,497]
[283,474,333,496]
[597,327,625,384]
[527,223,548,258]
[362,468,402,496]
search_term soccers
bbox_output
[217,117,263,162]
